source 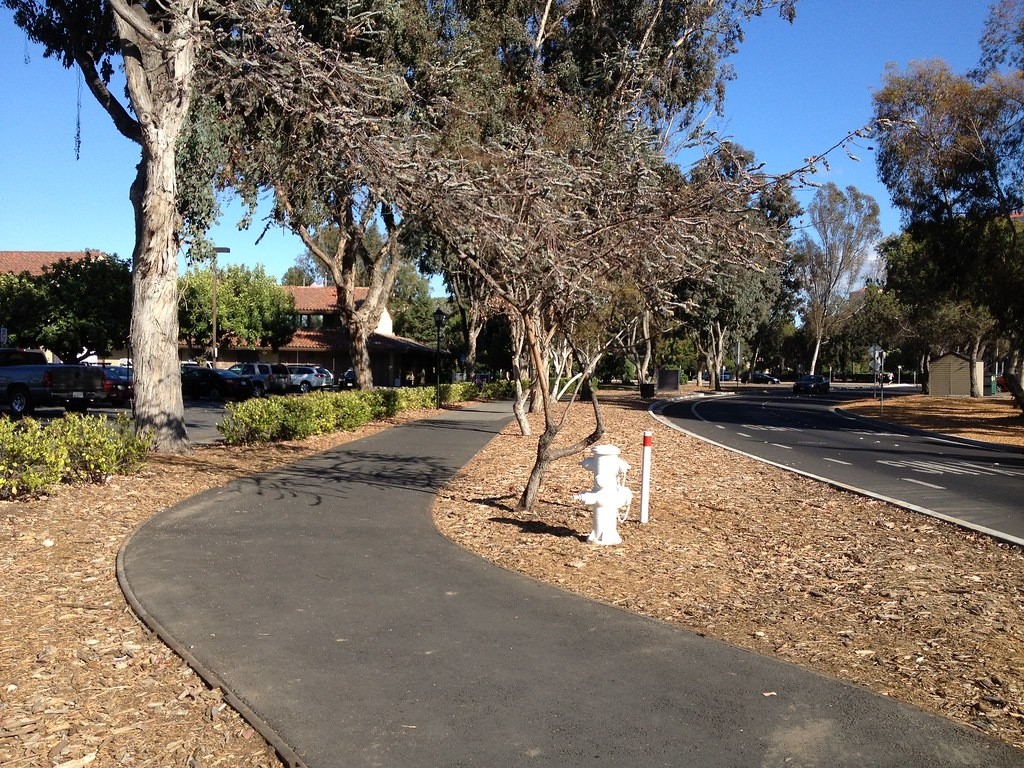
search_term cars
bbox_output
[339,368,360,391]
[793,375,831,394]
[693,372,734,381]
[180,365,256,402]
[91,364,135,406]
[741,372,779,385]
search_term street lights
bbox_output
[211,246,232,370]
[433,307,446,410]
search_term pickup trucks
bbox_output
[0,347,106,417]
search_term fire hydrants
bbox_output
[576,443,633,549]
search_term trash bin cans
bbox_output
[640,384,655,398]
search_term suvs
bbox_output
[226,362,293,399]
[286,364,334,394]
